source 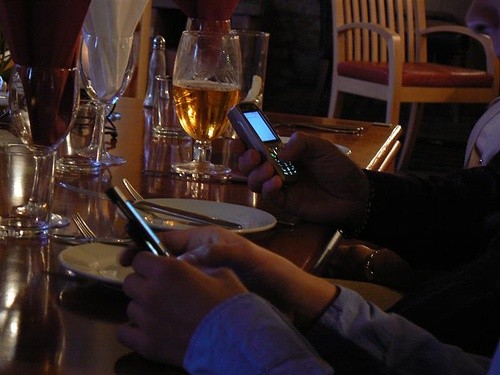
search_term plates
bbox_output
[56,240,149,287]
[129,196,277,235]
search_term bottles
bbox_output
[144,35,166,108]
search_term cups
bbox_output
[1,141,57,235]
[152,73,191,142]
[61,98,108,169]
[229,30,271,126]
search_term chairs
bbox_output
[329,1,499,170]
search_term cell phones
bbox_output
[105,185,171,256]
[226,101,301,179]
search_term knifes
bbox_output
[58,182,243,230]
[260,118,365,137]
[47,233,137,251]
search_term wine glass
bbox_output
[170,29,243,183]
[13,61,81,229]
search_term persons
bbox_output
[115,223,499,375]
[236,0,500,334]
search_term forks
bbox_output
[93,246,93,253]
[72,211,144,241]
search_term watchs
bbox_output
[359,247,383,280]
